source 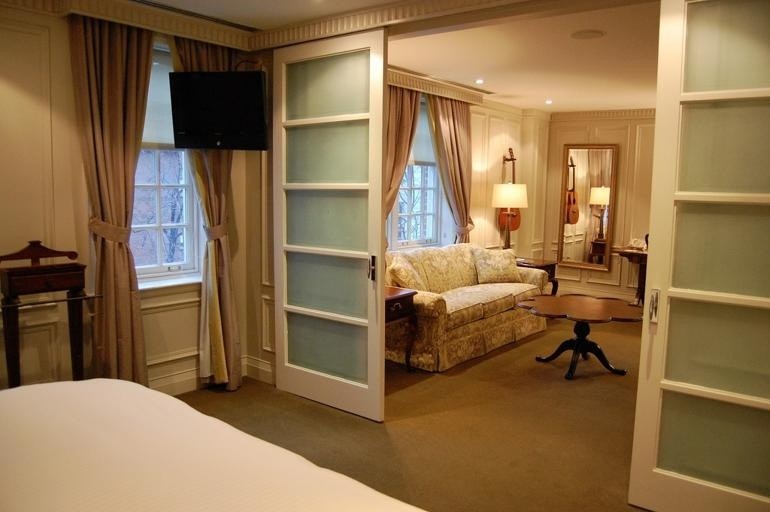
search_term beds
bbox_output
[1,371,430,512]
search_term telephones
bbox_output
[629,239,646,249]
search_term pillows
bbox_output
[388,255,427,292]
[470,245,524,284]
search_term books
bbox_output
[611,246,643,252]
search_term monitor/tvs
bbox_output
[168,70,271,149]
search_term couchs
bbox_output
[386,243,551,375]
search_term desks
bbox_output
[588,240,607,265]
[384,285,419,372]
[512,254,560,296]
[615,246,648,304]
[517,292,645,380]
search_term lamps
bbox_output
[589,185,611,239]
[492,181,529,250]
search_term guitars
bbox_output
[566,156,579,224]
[499,147,521,230]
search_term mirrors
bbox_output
[554,142,620,274]
